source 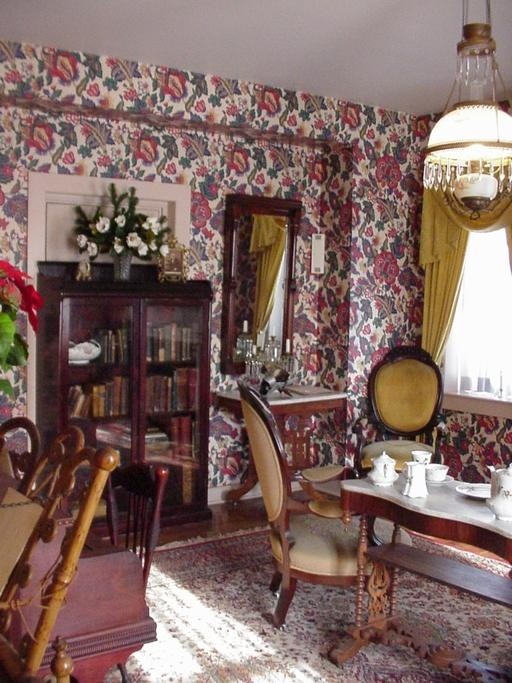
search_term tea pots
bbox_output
[367,451,399,487]
[485,463,512,521]
[401,462,428,497]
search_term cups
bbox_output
[425,464,449,481]
[410,451,432,465]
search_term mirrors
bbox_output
[222,193,301,375]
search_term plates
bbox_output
[456,483,493,498]
[427,475,454,488]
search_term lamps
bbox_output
[422,0,512,210]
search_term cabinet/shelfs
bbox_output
[38,262,213,535]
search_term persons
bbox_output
[167,252,180,271]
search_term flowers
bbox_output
[70,189,182,261]
[1,261,42,372]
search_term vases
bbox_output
[110,251,133,283]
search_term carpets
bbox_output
[112,520,511,682]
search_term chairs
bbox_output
[236,377,391,624]
[106,460,169,593]
[326,472,512,682]
[360,345,444,475]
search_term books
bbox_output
[145,365,198,413]
[91,375,130,417]
[95,419,170,460]
[146,321,200,362]
[157,463,201,505]
[67,381,91,420]
[159,413,200,462]
[91,324,130,366]
